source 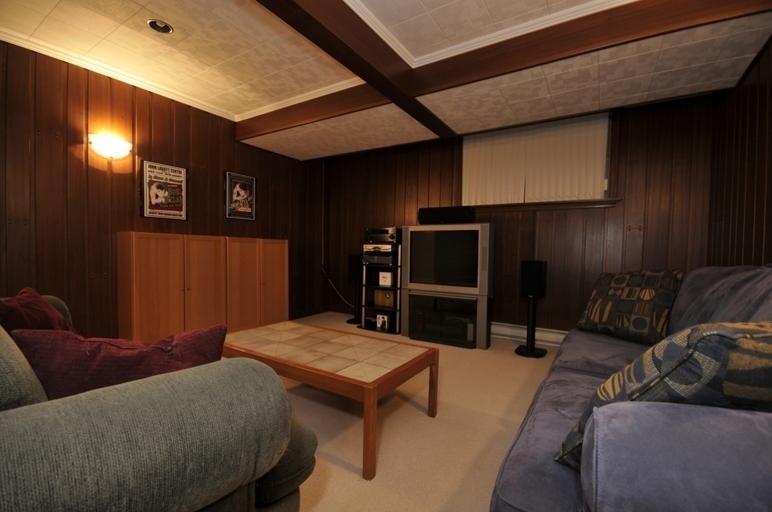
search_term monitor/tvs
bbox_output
[401,222,495,298]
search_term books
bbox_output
[367,255,392,265]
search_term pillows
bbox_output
[7,318,228,406]
[548,317,770,475]
[0,283,80,338]
[572,269,688,347]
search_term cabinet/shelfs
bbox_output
[219,234,289,335]
[360,242,403,332]
[116,229,226,347]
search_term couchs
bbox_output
[1,291,320,511]
[490,258,770,511]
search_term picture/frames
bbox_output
[223,169,257,223]
[138,159,188,223]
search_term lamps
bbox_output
[86,132,134,160]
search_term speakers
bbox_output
[520,260,547,298]
[348,255,363,285]
[416,207,476,224]
